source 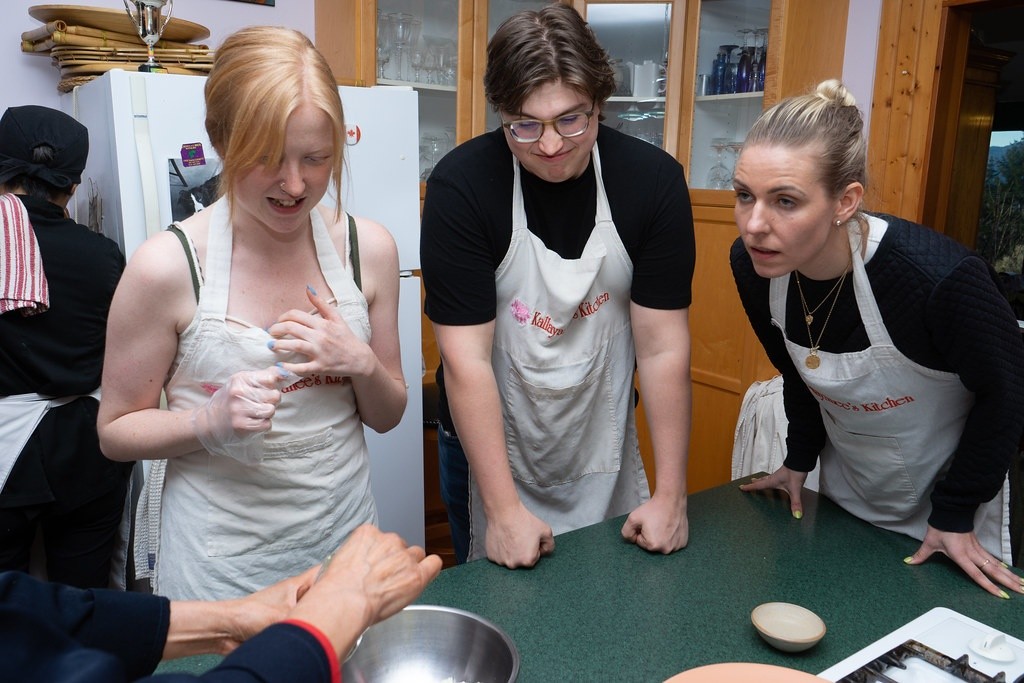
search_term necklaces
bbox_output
[794,260,851,369]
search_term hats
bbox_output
[0,104,88,188]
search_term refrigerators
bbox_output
[61,67,424,556]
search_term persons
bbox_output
[728,78,1024,600]
[0,525,443,683]
[96,25,407,599]
[0,105,138,592]
[420,0,697,569]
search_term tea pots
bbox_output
[627,59,667,97]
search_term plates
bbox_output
[661,662,834,683]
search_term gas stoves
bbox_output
[814,607,1024,683]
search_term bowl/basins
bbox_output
[750,602,826,650]
[340,602,520,683]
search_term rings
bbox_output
[980,559,990,568]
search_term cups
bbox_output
[696,73,713,96]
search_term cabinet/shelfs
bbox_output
[316,0,851,512]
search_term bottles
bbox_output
[711,54,727,94]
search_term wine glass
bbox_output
[722,27,768,92]
[375,8,457,87]
[706,143,743,190]
[421,132,456,180]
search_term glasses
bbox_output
[499,91,596,142]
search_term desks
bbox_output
[159,472,1024,683]
